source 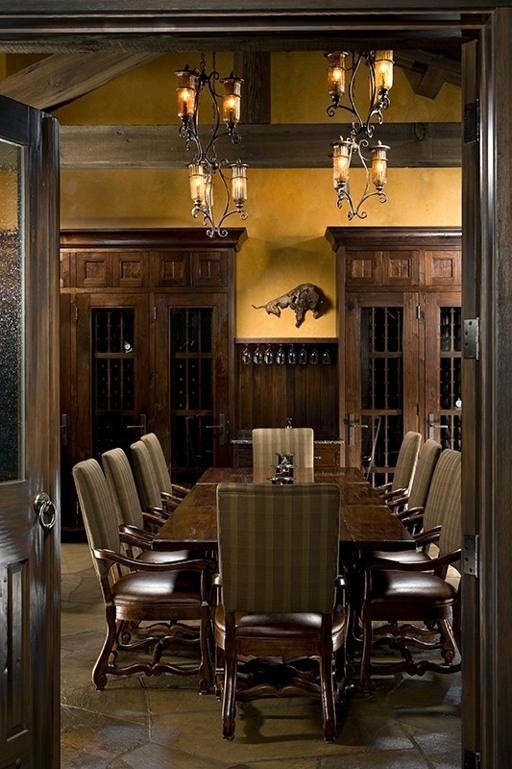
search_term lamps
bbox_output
[325,48,396,222]
[176,49,248,237]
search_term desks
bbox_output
[150,464,425,701]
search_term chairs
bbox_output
[248,429,317,469]
[210,480,355,743]
[100,429,190,537]
[72,454,220,696]
[374,430,460,538]
[343,463,462,704]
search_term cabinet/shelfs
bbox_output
[58,227,247,544]
[325,225,463,481]
